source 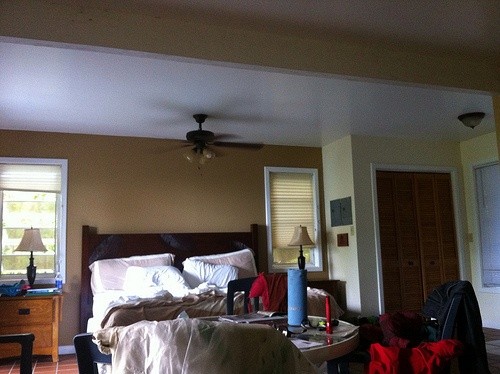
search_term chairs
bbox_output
[226,273,288,315]
[327,280,491,374]
[73,319,322,374]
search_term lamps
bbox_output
[288,223,314,270]
[183,144,215,163]
[458,112,485,130]
[14,227,48,289]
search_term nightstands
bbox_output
[306,279,346,308]
[0,288,64,363]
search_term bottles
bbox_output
[55,272,63,289]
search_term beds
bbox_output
[79,223,345,336]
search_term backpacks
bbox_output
[423,280,493,374]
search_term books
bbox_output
[302,326,360,337]
[218,310,277,323]
[291,338,324,349]
[24,288,63,296]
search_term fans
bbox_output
[149,113,264,157]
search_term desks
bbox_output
[191,315,359,364]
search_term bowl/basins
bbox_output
[0,285,20,296]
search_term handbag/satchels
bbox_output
[360,306,455,374]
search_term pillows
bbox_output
[89,250,258,300]
